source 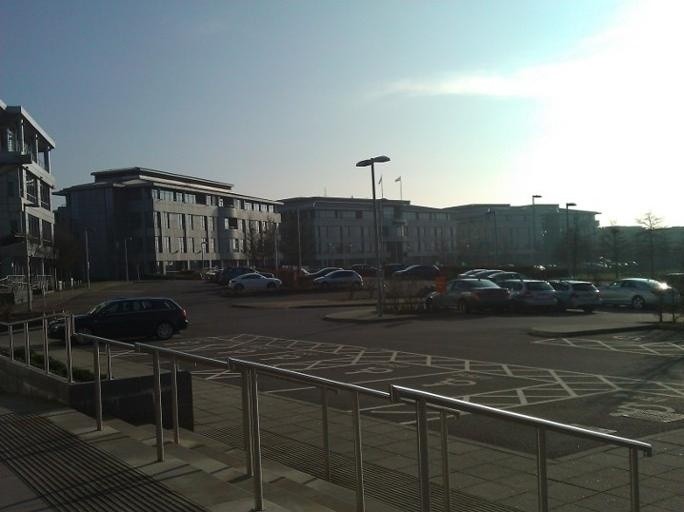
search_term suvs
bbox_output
[45,294,190,346]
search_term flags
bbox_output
[395,176,401,181]
[379,176,382,184]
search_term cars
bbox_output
[596,276,681,312]
[425,275,512,315]
[658,273,684,295]
[495,277,559,311]
[548,278,601,314]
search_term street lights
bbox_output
[531,195,542,248]
[566,202,577,228]
[21,202,40,311]
[485,210,498,261]
[200,241,207,269]
[121,236,134,280]
[355,154,390,311]
